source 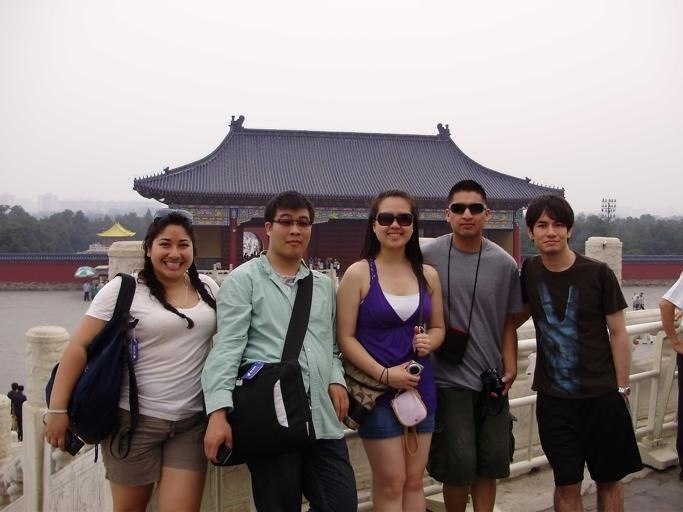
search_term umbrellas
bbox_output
[74,265,97,279]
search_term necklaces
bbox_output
[163,273,190,309]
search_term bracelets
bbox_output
[377,367,386,385]
[386,367,389,387]
[45,409,68,414]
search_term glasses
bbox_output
[376,213,413,227]
[449,203,487,215]
[271,219,310,227]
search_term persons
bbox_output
[336,190,445,512]
[632,291,646,310]
[421,178,523,512]
[42,211,221,512]
[306,255,341,277]
[660,271,683,483]
[200,190,358,512]
[82,278,109,303]
[7,382,27,443]
[514,194,644,511]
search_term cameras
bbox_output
[480,367,505,393]
[406,359,425,376]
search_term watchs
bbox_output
[618,387,633,397]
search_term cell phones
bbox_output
[218,444,233,467]
[64,428,85,457]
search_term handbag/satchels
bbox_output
[211,361,310,467]
[46,273,135,445]
[339,351,387,431]
